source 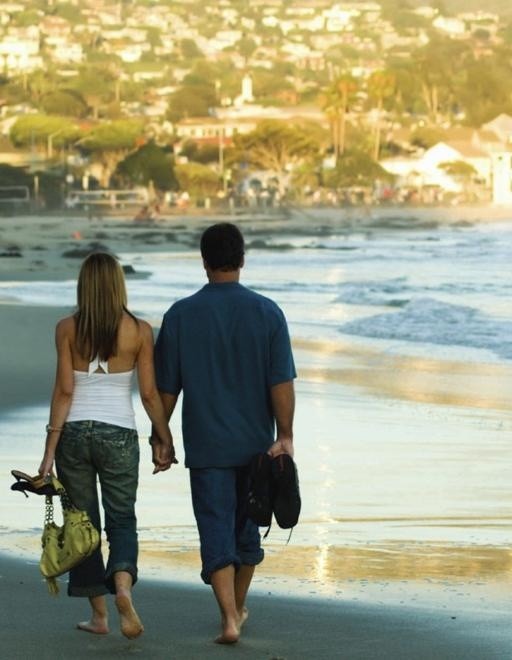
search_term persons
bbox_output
[150,223,297,644]
[39,252,175,640]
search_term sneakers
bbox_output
[243,454,301,529]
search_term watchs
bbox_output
[46,424,64,433]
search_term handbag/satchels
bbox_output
[38,473,100,579]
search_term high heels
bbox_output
[10,470,60,498]
